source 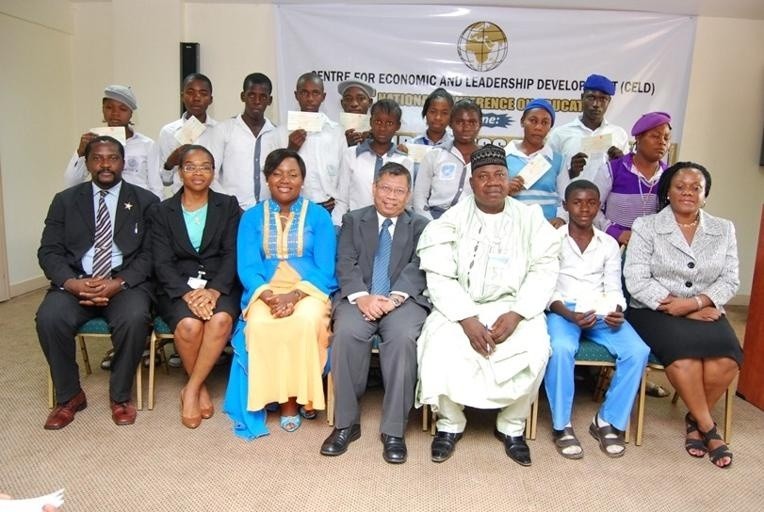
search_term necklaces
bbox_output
[677,215,699,228]
[181,203,207,226]
[635,162,660,217]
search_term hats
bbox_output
[100,83,137,112]
[582,74,616,95]
[658,161,712,206]
[522,97,555,128]
[336,78,376,97]
[181,164,212,174]
[469,142,508,175]
[630,111,671,138]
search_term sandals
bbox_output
[637,380,670,397]
[299,404,316,419]
[550,419,584,459]
[698,421,734,468]
[587,413,626,459]
[684,410,710,459]
[278,412,301,432]
[98,346,235,371]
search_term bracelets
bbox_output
[694,295,703,311]
[289,289,302,299]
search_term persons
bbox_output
[621,160,745,469]
[540,179,652,462]
[258,71,348,216]
[590,110,673,399]
[328,99,411,229]
[410,143,557,468]
[317,161,432,464]
[205,73,276,212]
[411,99,485,222]
[148,145,241,432]
[62,83,162,372]
[395,87,455,157]
[499,97,567,229]
[334,78,377,148]
[152,71,218,367]
[218,146,337,441]
[548,73,631,209]
[32,133,160,432]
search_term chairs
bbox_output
[47,317,142,410]
[621,245,736,445]
[149,244,530,441]
[531,337,630,443]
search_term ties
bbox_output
[369,218,394,297]
[90,190,113,280]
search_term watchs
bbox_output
[116,276,127,289]
[389,296,401,307]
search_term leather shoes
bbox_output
[430,431,463,463]
[318,422,362,456]
[492,427,533,467]
[377,426,408,464]
[178,385,202,429]
[109,391,137,426]
[199,404,214,420]
[42,387,87,431]
